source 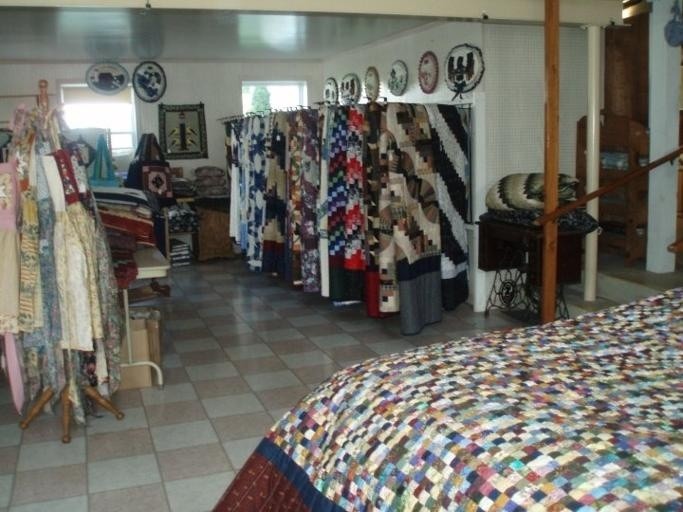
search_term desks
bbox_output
[113,243,174,390]
[478,209,573,325]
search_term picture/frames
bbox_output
[157,101,210,162]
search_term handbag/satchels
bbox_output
[88,138,121,187]
[127,134,176,205]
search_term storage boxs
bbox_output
[113,304,162,390]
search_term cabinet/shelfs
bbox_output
[577,116,650,273]
